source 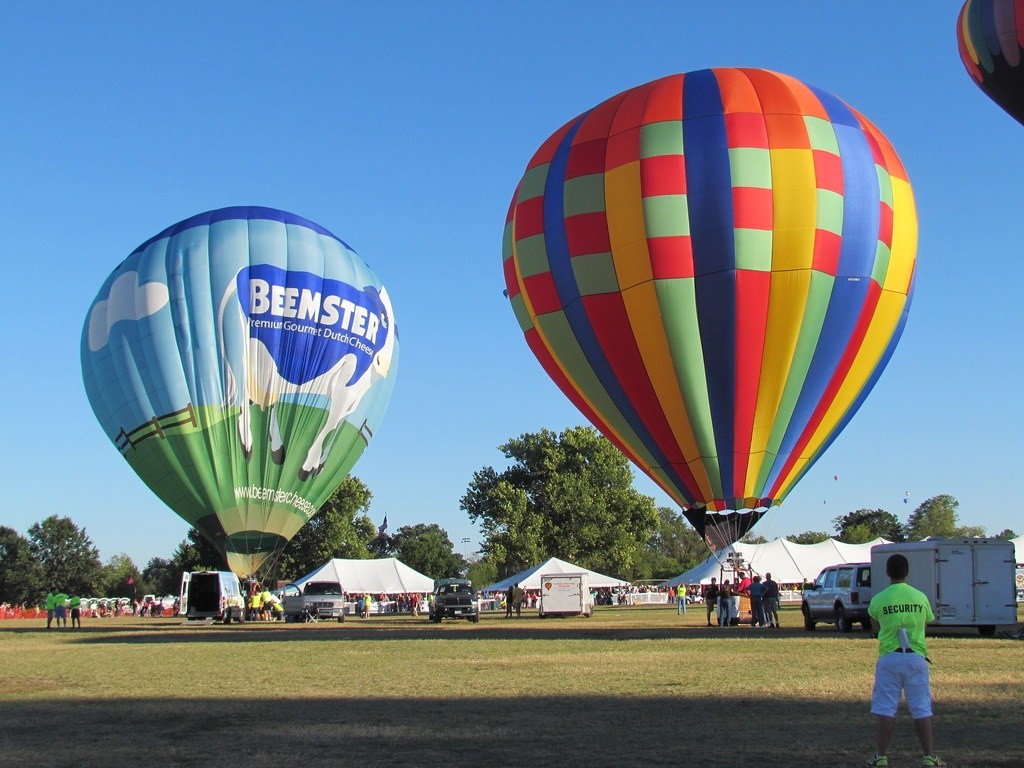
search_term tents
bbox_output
[481,557,633,592]
[272,556,435,594]
[974,533,1024,564]
[662,536,934,582]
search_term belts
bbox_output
[895,648,933,666]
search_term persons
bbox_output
[344,592,436,618]
[703,578,719,627]
[505,586,514,618]
[476,590,541,609]
[732,577,747,594]
[677,583,687,615]
[596,588,613,605]
[45,586,58,628]
[740,572,780,628]
[0,597,179,616]
[70,593,81,628]
[733,572,752,596]
[801,578,807,596]
[512,583,525,617]
[719,580,734,616]
[613,585,696,605]
[786,583,799,590]
[864,553,948,767]
[243,583,285,625]
[53,589,70,628]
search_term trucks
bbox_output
[539,572,593,619]
[870,535,1018,636]
[178,570,244,624]
[429,579,481,623]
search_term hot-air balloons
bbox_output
[834,475,839,481]
[79,205,399,622]
[503,67,918,622]
[903,491,910,504]
[957,0,1024,128]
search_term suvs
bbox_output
[801,562,872,632]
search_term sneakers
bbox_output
[866,753,887,768]
[923,753,946,768]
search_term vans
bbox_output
[283,580,346,623]
